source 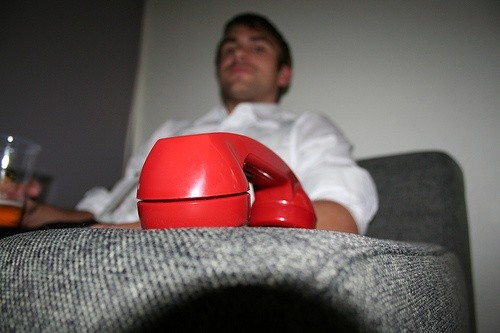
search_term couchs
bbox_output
[0,150,478,333]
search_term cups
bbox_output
[0,135,43,229]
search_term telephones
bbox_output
[135,133,318,229]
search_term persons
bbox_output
[21,12,379,235]
